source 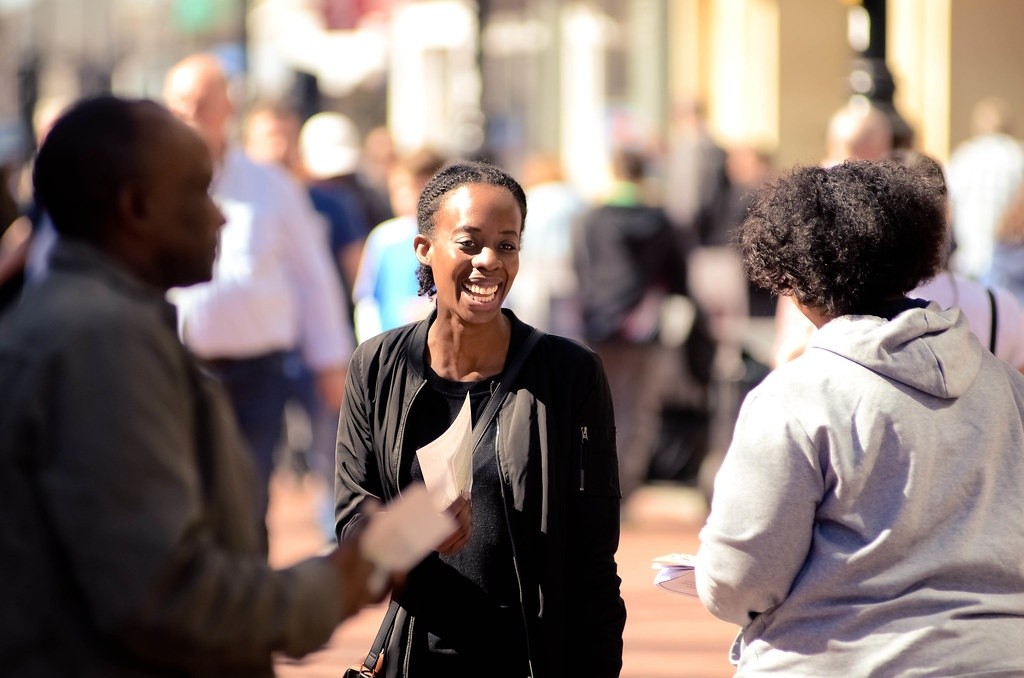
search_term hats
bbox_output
[297,112,360,180]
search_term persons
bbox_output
[573,147,686,503]
[350,147,448,343]
[160,55,356,562]
[500,155,595,345]
[0,54,1024,393]
[298,111,395,329]
[693,161,1024,677]
[0,96,466,678]
[333,165,628,678]
[241,109,364,483]
